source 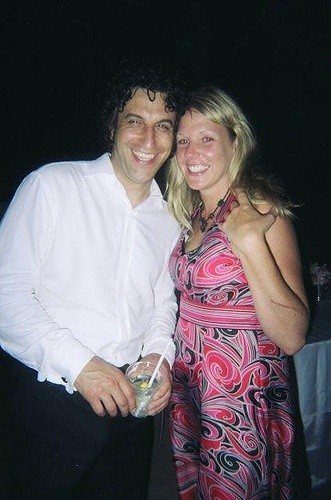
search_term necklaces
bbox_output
[200,185,231,233]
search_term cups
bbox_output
[124,361,166,419]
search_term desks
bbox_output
[291,298,331,500]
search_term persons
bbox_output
[0,62,194,500]
[151,83,314,499]
